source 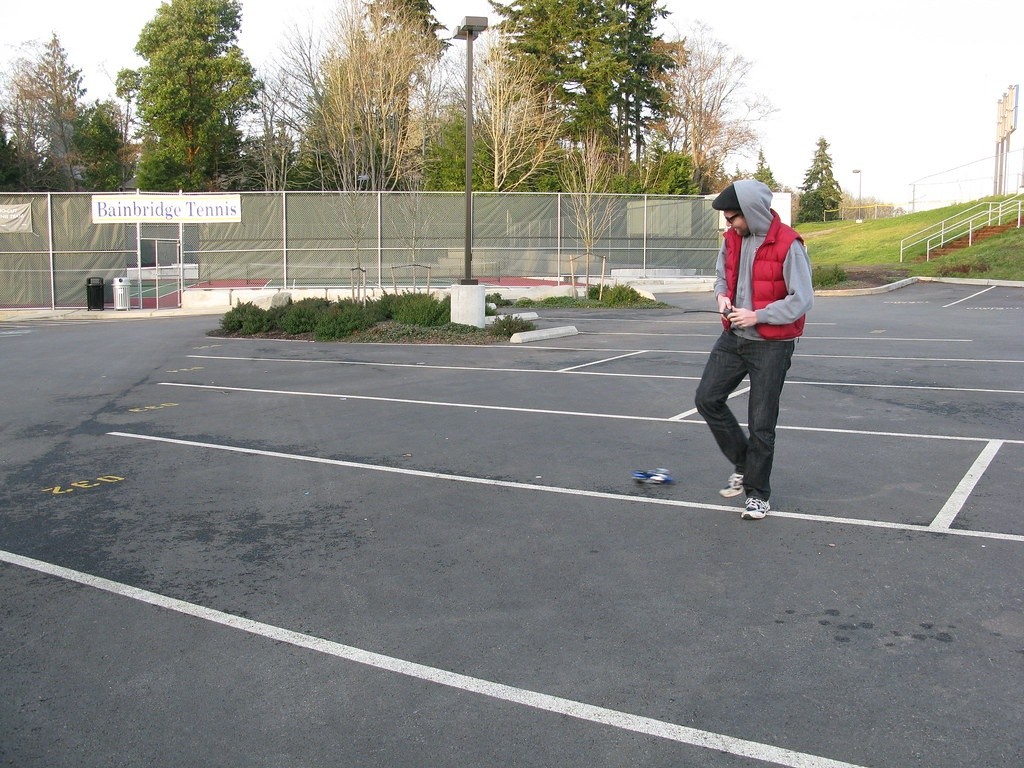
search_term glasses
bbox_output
[725,213,742,224]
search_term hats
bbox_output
[712,184,740,210]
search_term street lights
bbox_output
[853,169,862,223]
[453,16,489,284]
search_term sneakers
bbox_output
[741,497,771,520]
[719,473,743,498]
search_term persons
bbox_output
[694,179,815,521]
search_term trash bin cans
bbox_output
[85,277,105,311]
[111,277,132,311]
